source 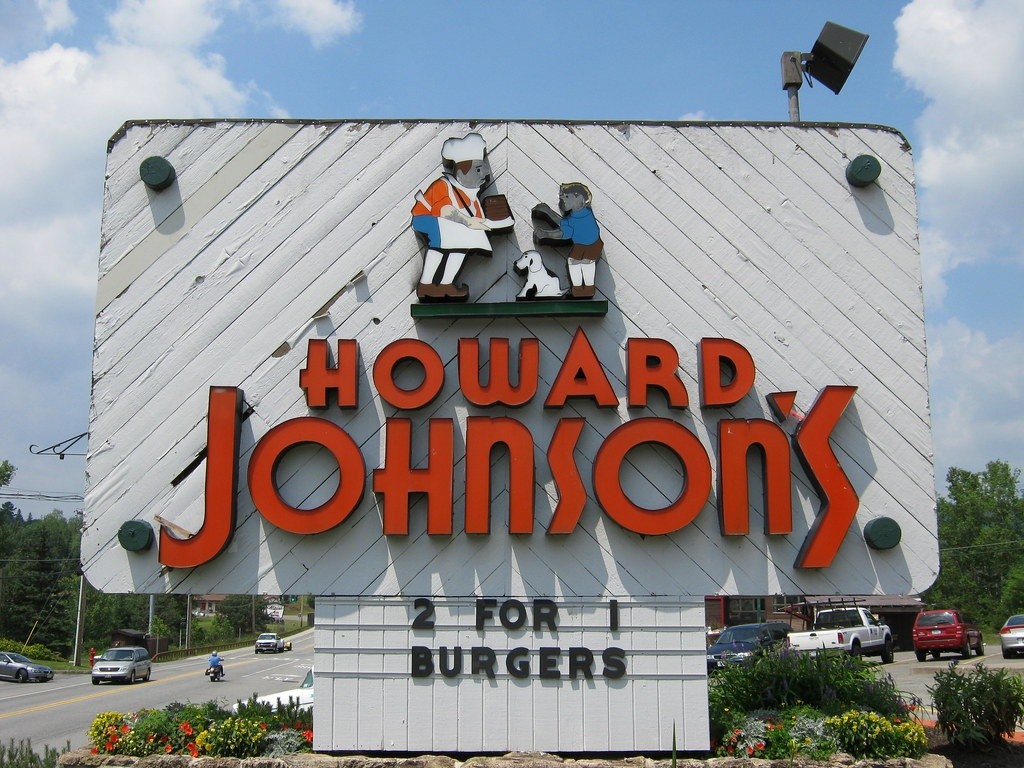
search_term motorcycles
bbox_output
[208,668,220,682]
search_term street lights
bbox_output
[780,20,869,123]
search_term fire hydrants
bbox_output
[90,647,95,668]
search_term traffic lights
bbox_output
[290,597,297,602]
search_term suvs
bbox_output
[92,647,152,684]
[913,609,984,662]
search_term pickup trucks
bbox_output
[255,633,284,654]
[787,607,893,663]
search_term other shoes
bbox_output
[221,674,225,677]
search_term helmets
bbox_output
[212,650,217,656]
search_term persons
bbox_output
[208,651,224,678]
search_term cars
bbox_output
[0,652,53,683]
[999,615,1024,659]
[707,622,793,678]
